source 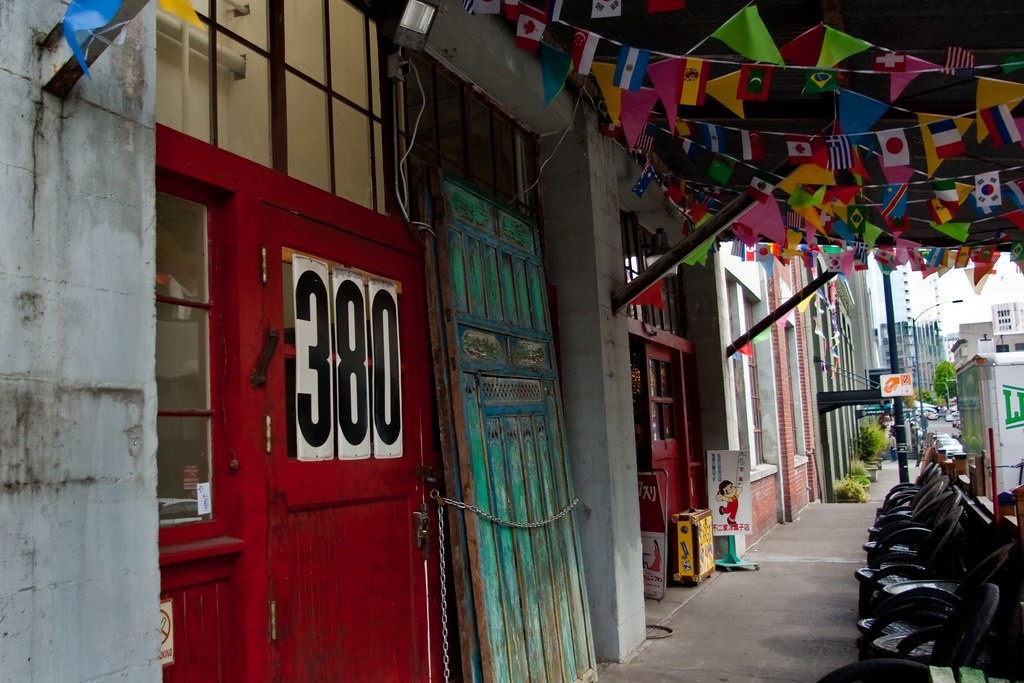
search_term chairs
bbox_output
[816,460,1019,683]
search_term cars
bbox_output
[902,397,964,462]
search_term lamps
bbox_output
[349,1,438,53]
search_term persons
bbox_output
[888,432,898,462]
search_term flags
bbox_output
[999,212,1024,231]
[978,104,1021,147]
[701,122,724,152]
[824,134,855,169]
[932,176,961,211]
[880,182,908,219]
[1003,178,1024,211]
[634,121,660,156]
[736,64,774,101]
[785,204,802,229]
[590,0,621,18]
[874,50,907,71]
[600,114,621,138]
[683,138,696,155]
[973,170,1001,208]
[746,169,780,207]
[875,129,910,168]
[570,29,599,76]
[630,159,655,199]
[515,2,548,54]
[674,118,691,136]
[802,65,840,93]
[612,46,648,94]
[739,130,765,160]
[926,184,972,224]
[664,174,722,235]
[927,117,966,160]
[649,0,683,12]
[846,205,868,234]
[679,58,709,107]
[884,213,912,233]
[784,133,814,165]
[965,190,1002,216]
[1001,56,1024,73]
[941,46,975,77]
[732,221,1024,276]
[708,153,737,186]
[813,206,833,233]
[462,0,519,20]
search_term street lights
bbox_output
[910,299,963,436]
